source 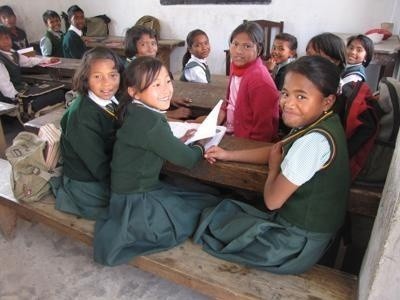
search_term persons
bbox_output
[1,5,87,117]
[49,21,375,273]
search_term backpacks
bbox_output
[136,15,160,39]
[342,77,400,182]
[84,15,110,36]
[5,131,63,201]
[16,83,65,125]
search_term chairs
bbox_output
[224,20,284,75]
